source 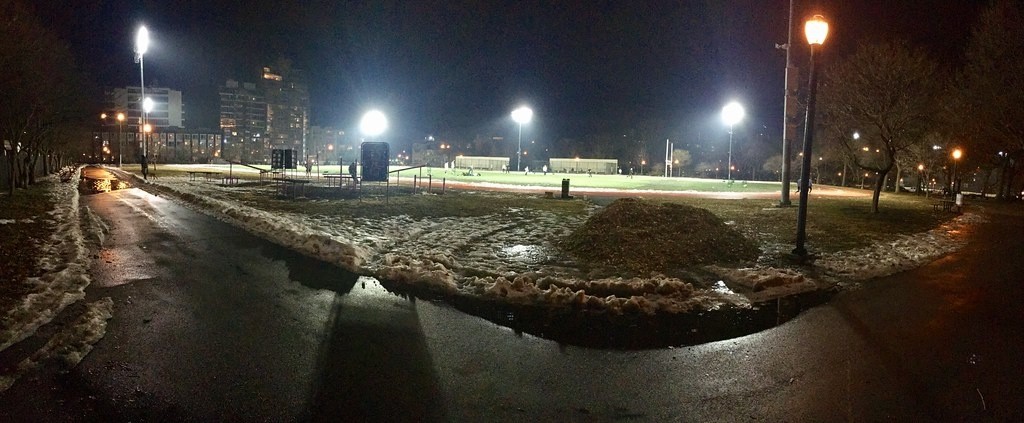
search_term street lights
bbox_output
[134,25,150,156]
[720,100,746,180]
[784,13,830,261]
[117,113,125,164]
[951,148,962,192]
[510,106,533,169]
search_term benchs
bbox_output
[219,177,242,185]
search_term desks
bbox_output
[186,171,220,183]
[258,169,283,181]
[933,200,960,214]
[324,176,361,192]
[272,177,311,201]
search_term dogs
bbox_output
[150,173,158,181]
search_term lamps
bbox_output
[804,14,830,47]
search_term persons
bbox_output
[808,179,813,193]
[446,159,634,178]
[140,155,147,180]
[795,177,800,194]
[297,159,318,176]
